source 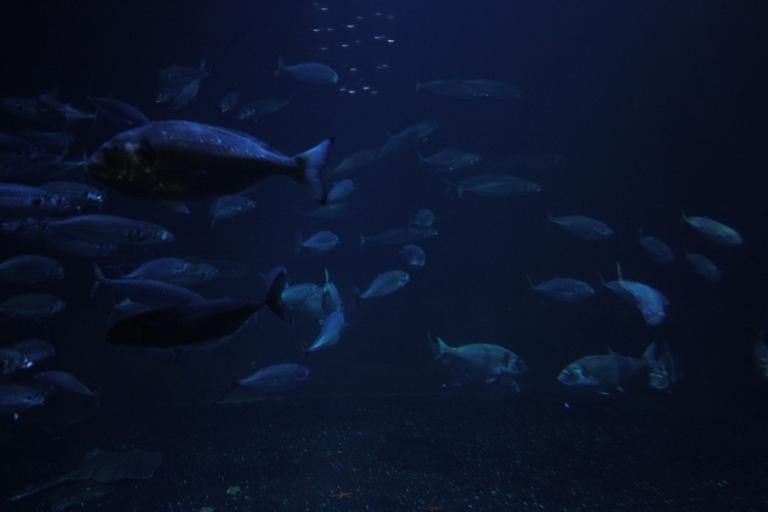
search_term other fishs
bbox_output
[1,55,356,414]
